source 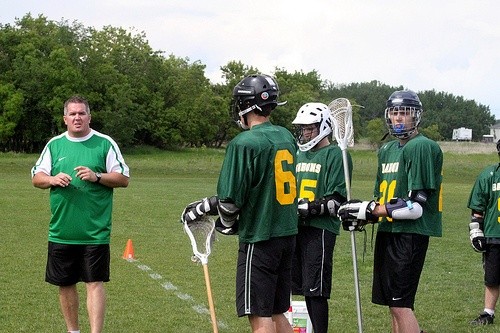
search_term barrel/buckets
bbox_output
[283,301,313,333]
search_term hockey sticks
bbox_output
[327,97,363,333]
[182,214,220,333]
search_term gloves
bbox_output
[336,199,378,230]
[469,221,487,252]
[214,216,240,235]
[180,195,219,224]
[297,197,317,218]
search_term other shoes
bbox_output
[472,310,494,325]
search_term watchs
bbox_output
[91,173,101,185]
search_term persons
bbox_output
[180,74,298,333]
[30,94,130,333]
[289,102,354,333]
[337,90,444,333]
[467,140,500,325]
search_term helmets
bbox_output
[291,103,334,152]
[386,90,422,109]
[232,74,279,110]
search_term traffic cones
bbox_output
[123,239,135,260]
